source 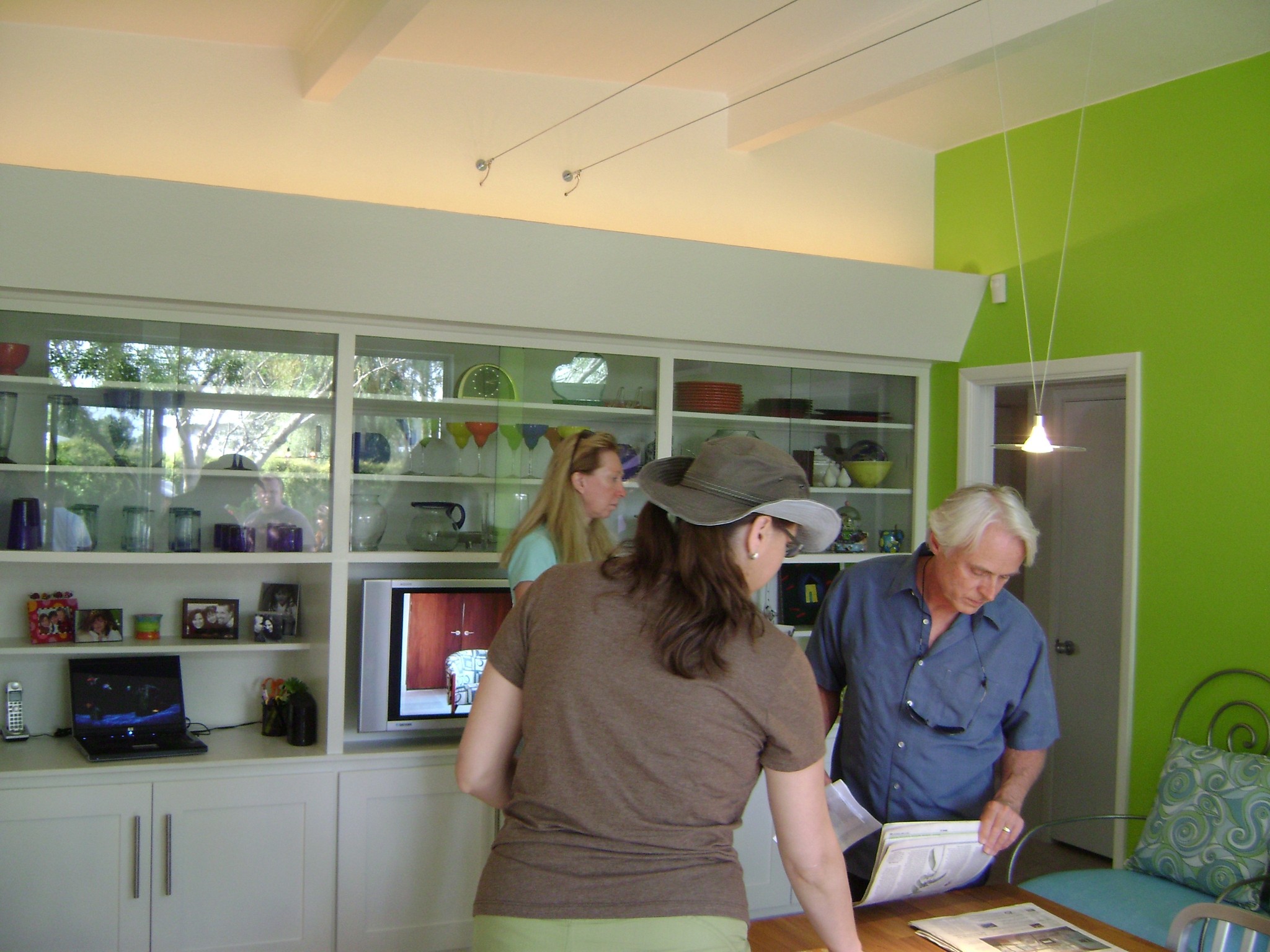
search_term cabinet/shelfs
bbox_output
[0,295,935,952]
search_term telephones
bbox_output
[1,681,30,741]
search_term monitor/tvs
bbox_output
[357,579,513,732]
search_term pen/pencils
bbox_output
[265,685,287,734]
[982,680,986,688]
[262,688,269,705]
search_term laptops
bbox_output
[68,655,208,762]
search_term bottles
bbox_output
[824,461,851,487]
[353,494,387,552]
[288,692,316,747]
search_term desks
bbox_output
[749,881,1173,952]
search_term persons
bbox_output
[187,602,235,638]
[172,438,484,555]
[254,584,298,641]
[77,610,123,642]
[806,482,1062,901]
[38,607,71,634]
[454,435,864,952]
[499,429,627,608]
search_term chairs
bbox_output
[443,648,489,713]
[1166,902,1270,952]
[1008,668,1270,952]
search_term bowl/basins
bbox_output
[775,624,794,637]
[0,342,29,375]
[842,461,893,487]
[352,432,390,474]
[813,449,832,487]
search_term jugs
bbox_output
[406,502,465,551]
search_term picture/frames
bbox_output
[26,591,78,645]
[181,598,239,640]
[260,581,301,638]
[73,608,123,643]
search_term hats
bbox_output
[638,433,843,555]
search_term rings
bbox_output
[1002,826,1011,833]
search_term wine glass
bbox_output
[102,386,187,468]
[498,424,549,479]
[445,423,472,477]
[396,417,436,476]
[465,421,498,477]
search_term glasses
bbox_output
[781,527,805,557]
[570,429,596,470]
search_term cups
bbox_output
[793,450,814,487]
[47,395,79,465]
[0,392,18,464]
[266,523,302,552]
[213,524,255,552]
[544,426,592,452]
[133,614,163,640]
[879,530,904,552]
[7,497,42,551]
[121,506,155,552]
[169,507,201,551]
[261,701,287,736]
[68,505,98,552]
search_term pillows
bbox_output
[1123,736,1270,911]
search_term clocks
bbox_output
[453,362,518,402]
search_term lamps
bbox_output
[983,0,1099,453]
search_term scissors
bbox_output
[263,677,285,698]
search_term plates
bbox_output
[755,398,894,422]
[675,381,745,412]
